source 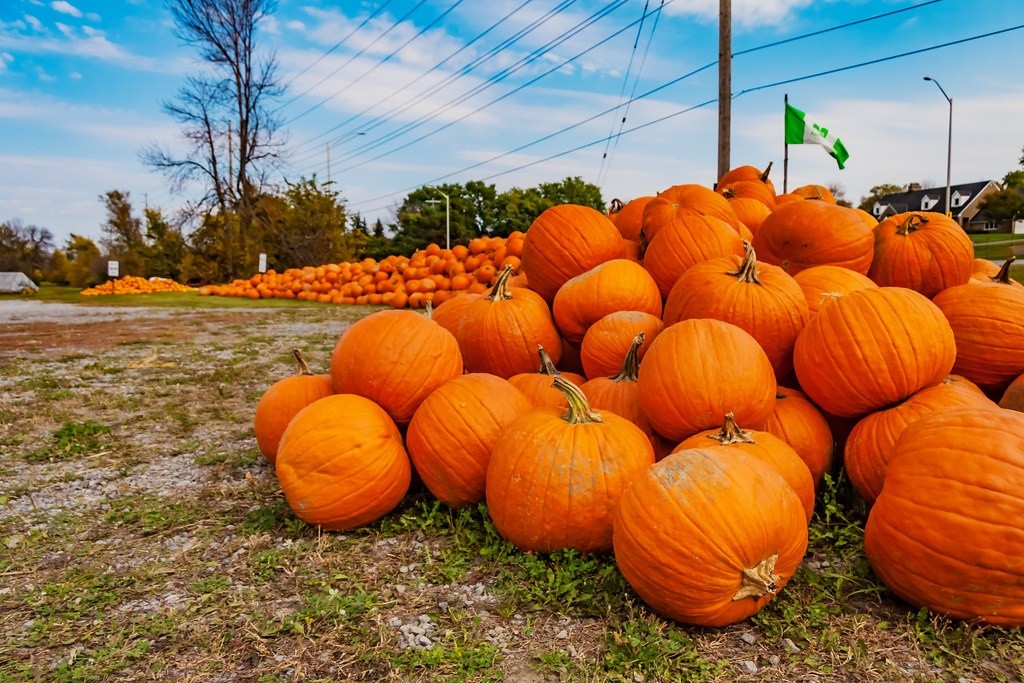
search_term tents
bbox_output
[0,271,39,294]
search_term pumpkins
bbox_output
[253,163,1024,628]
[80,231,526,309]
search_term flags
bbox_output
[785,101,850,170]
